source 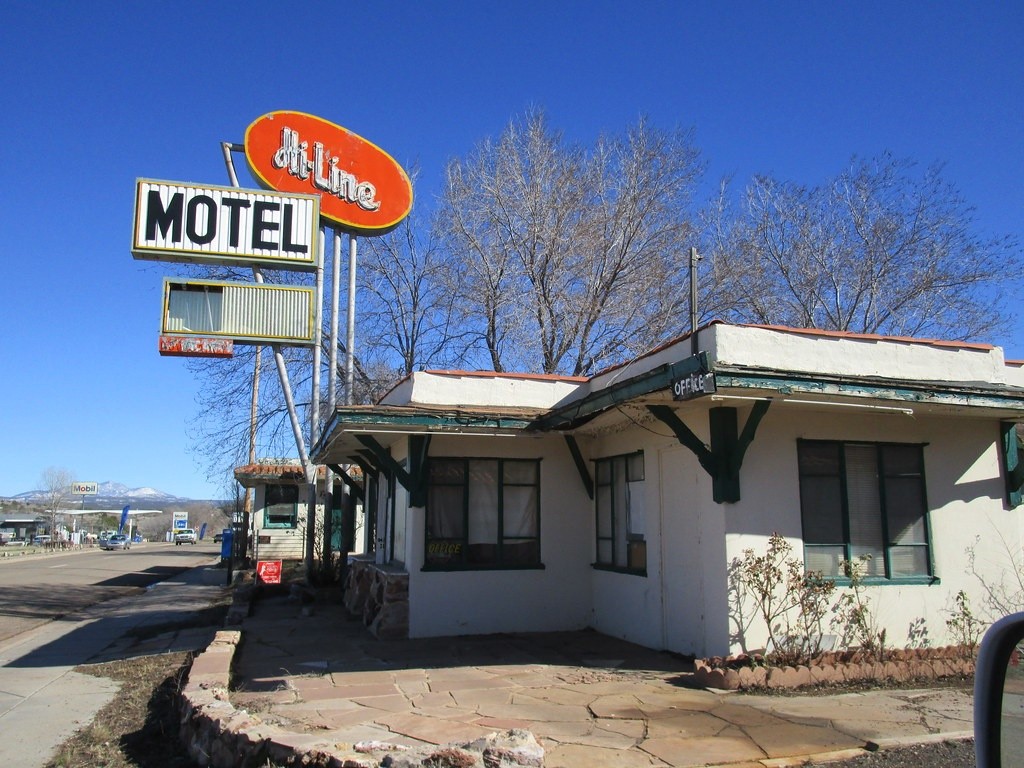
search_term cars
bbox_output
[99,535,130,551]
[213,534,224,543]
[174,529,198,544]
